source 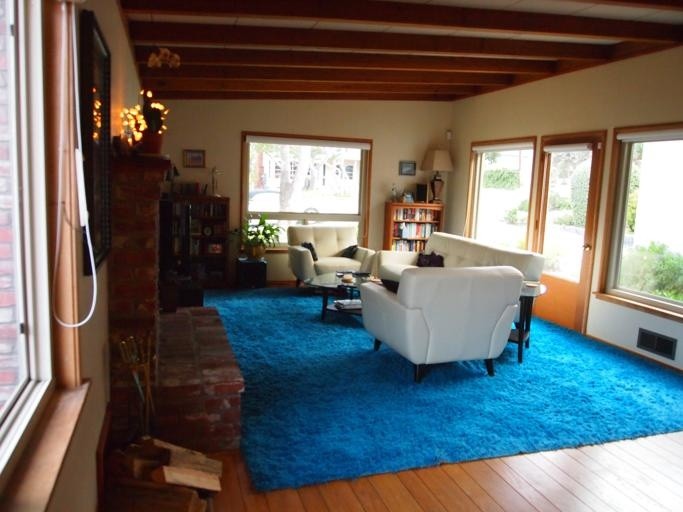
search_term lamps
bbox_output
[420,150,454,204]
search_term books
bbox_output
[392,208,438,252]
[172,201,225,256]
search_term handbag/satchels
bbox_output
[301,242,317,260]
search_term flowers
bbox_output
[143,47,181,134]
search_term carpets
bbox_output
[198,286,683,493]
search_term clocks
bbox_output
[202,225,213,236]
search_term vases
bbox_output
[141,128,163,154]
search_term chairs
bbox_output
[359,265,525,384]
[286,225,376,296]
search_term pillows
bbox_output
[340,245,358,258]
[381,279,399,293]
[301,242,318,261]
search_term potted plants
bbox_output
[230,211,286,259]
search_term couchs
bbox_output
[377,232,545,329]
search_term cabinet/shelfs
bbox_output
[159,195,230,289]
[235,257,268,289]
[383,199,446,252]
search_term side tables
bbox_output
[508,283,547,362]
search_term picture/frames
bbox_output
[399,161,416,176]
[182,148,205,167]
[79,10,112,275]
